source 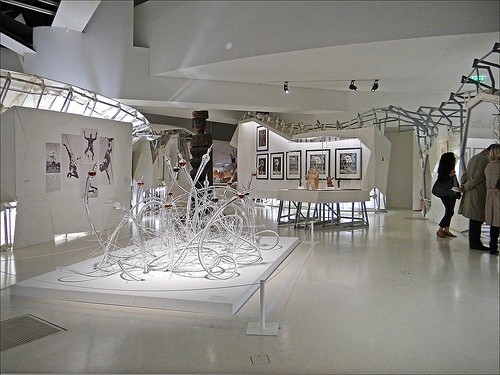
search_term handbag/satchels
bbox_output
[432,167,454,197]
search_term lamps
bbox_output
[284,81,290,94]
[349,80,358,91]
[371,79,379,92]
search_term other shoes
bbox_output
[437,229,457,237]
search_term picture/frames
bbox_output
[270,152,284,180]
[286,150,301,180]
[335,148,362,180]
[256,126,269,151]
[306,149,330,181]
[256,153,268,180]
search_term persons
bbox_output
[259,159,266,175]
[185,110,219,215]
[484,144,500,255]
[273,158,281,175]
[63,143,81,179]
[99,137,114,184]
[437,152,461,238]
[457,144,497,251]
[83,131,97,161]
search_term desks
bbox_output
[280,188,368,231]
